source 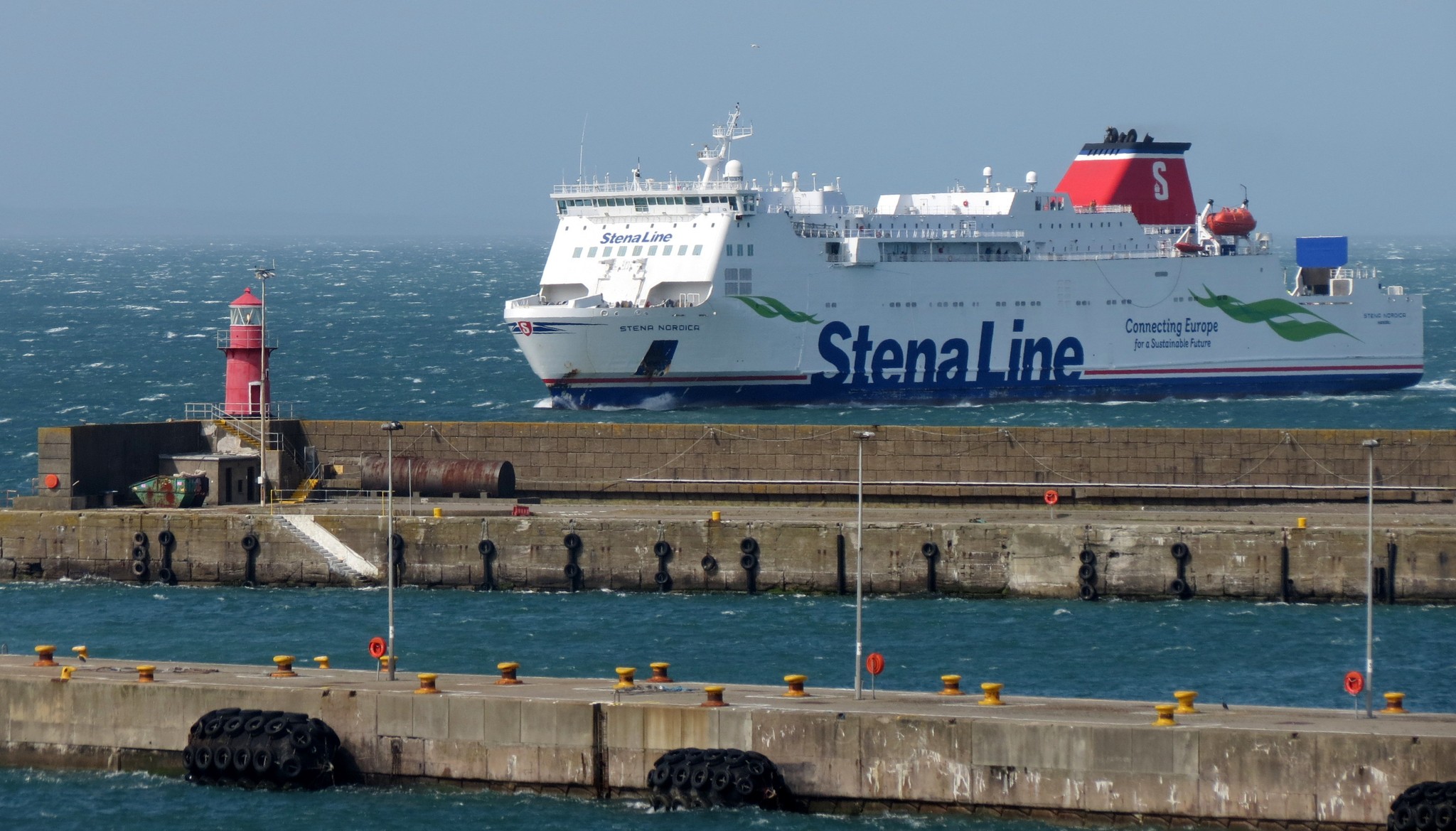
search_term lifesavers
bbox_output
[241,535,257,552]
[654,571,669,583]
[370,642,384,657]
[1170,579,1185,596]
[921,542,937,557]
[1044,490,1057,504]
[564,563,579,578]
[1387,780,1456,831]
[131,530,174,583]
[181,707,340,779]
[478,540,494,554]
[653,541,671,557]
[1170,542,1188,558]
[1078,549,1095,602]
[1346,677,1361,693]
[386,533,402,564]
[646,747,783,812]
[739,536,758,570]
[700,555,716,572]
[241,580,255,588]
[563,534,581,550]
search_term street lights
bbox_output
[850,430,875,701]
[1361,439,1381,719]
[248,265,276,508]
[380,421,404,682]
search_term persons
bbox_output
[1050,199,1056,210]
[1058,201,1062,210]
[985,246,1030,262]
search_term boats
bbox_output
[1207,206,1258,236]
[498,98,1427,414]
[1174,242,1204,253]
